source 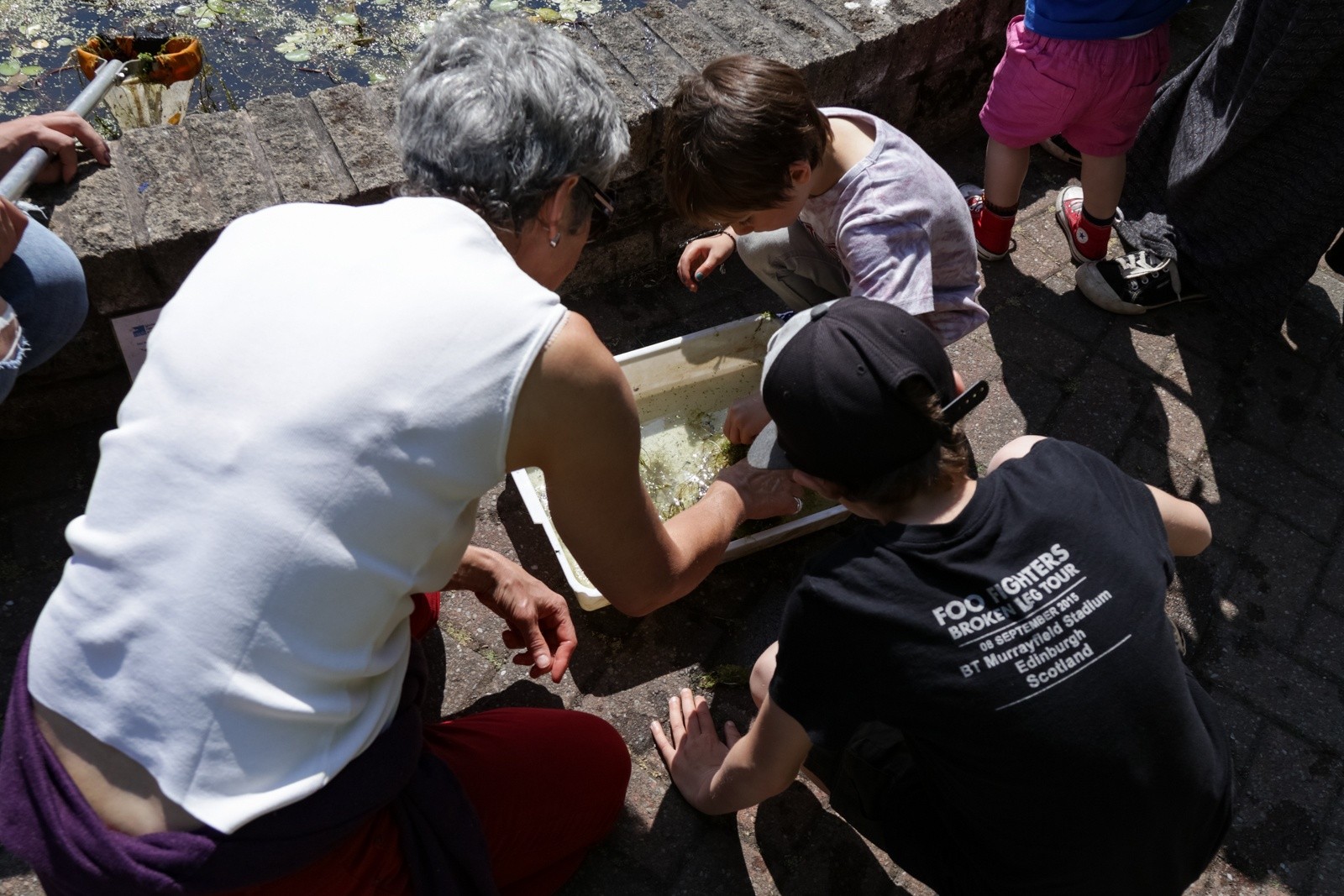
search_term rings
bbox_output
[794,496,807,516]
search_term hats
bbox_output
[748,293,989,470]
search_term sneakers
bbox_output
[957,182,1015,261]
[1056,185,1125,263]
[1039,133,1082,167]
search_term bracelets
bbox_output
[681,227,737,276]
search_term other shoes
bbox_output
[1076,250,1218,314]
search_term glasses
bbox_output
[543,172,615,244]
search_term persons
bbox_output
[1074,0,1344,315]
[0,7,815,896]
[1,110,119,594]
[650,296,1236,896]
[667,52,992,446]
[957,0,1179,261]
